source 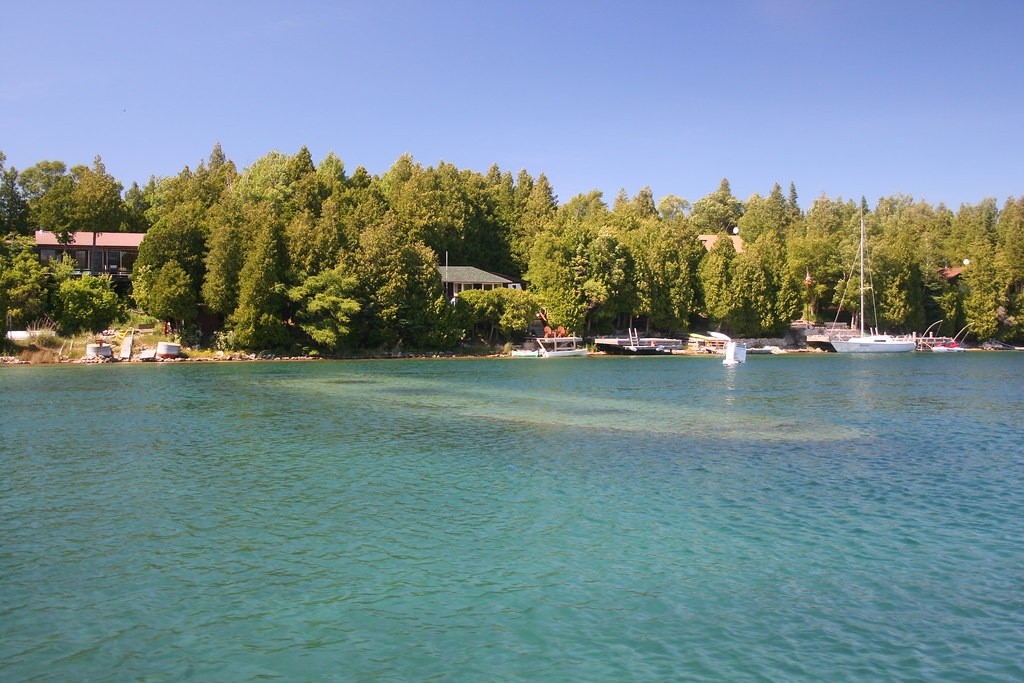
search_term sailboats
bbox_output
[806,214,917,352]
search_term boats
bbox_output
[538,335,590,358]
[931,346,965,354]
[593,328,684,355]
